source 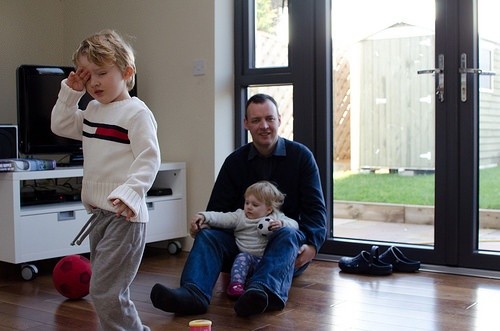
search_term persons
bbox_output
[49,26,162,331]
[149,94,328,318]
[190,180,300,295]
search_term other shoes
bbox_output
[231,282,243,293]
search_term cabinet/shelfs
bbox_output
[0,162,189,280]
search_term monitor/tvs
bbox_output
[16,64,137,167]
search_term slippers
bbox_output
[339,246,392,275]
[379,245,420,273]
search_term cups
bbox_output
[189,319,212,331]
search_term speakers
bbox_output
[0,123,18,159]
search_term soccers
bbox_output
[257,217,275,238]
[52,255,92,300]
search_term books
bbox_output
[1,158,58,172]
